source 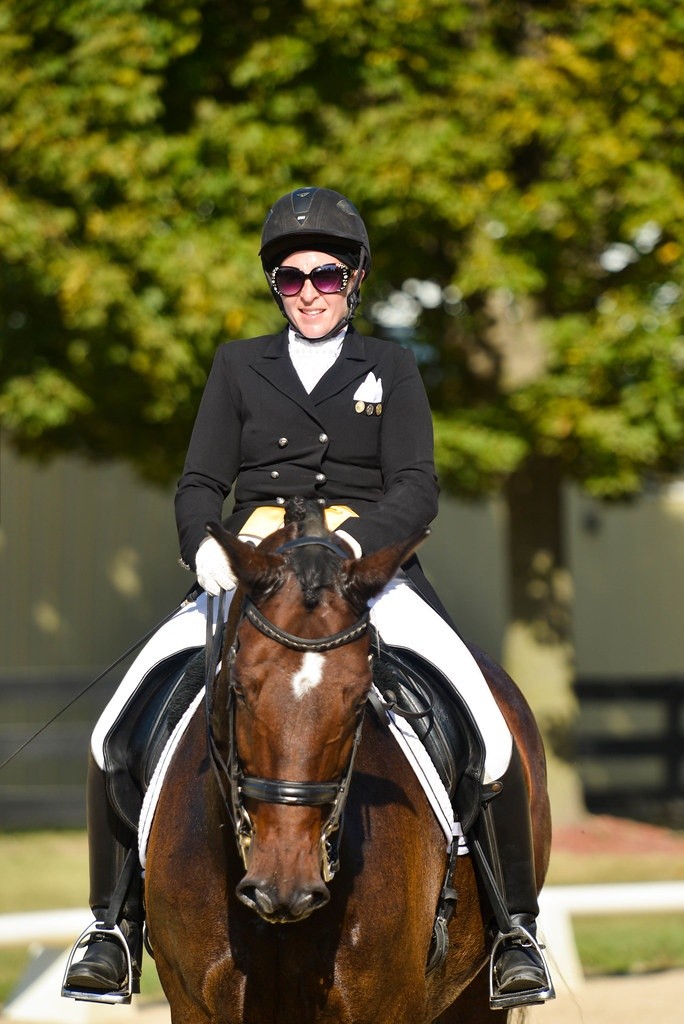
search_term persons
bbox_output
[67,188,557,1004]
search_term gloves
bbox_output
[333,530,361,560]
[194,533,237,596]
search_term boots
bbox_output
[65,744,143,995]
[470,735,547,1008]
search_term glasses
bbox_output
[269,263,356,296]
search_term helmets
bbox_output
[258,186,372,279]
